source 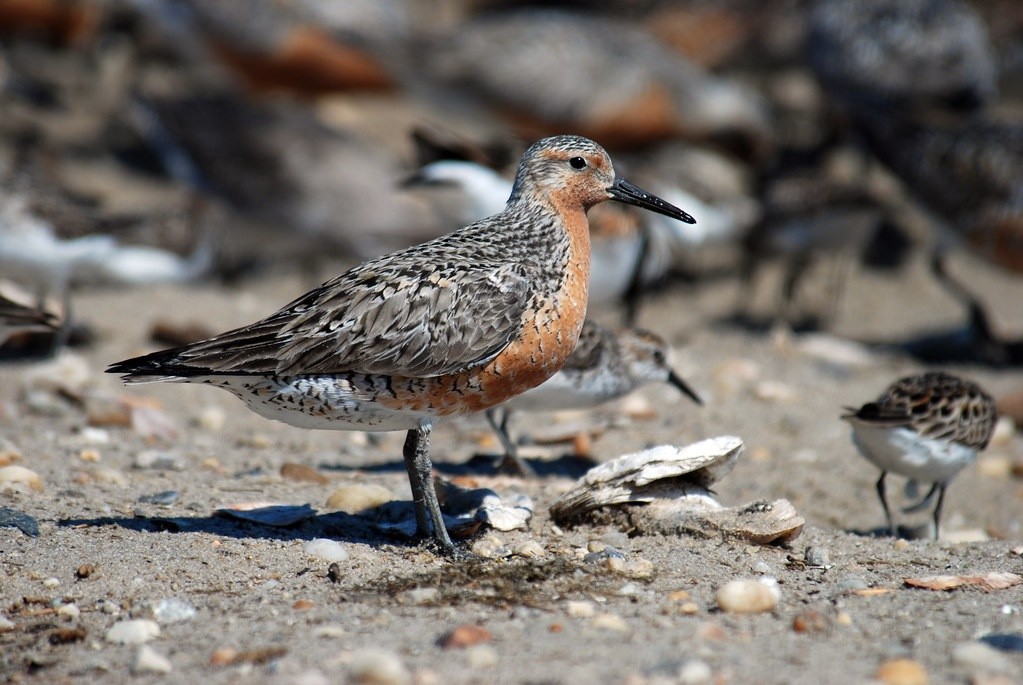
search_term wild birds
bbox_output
[0,0,1023,566]
